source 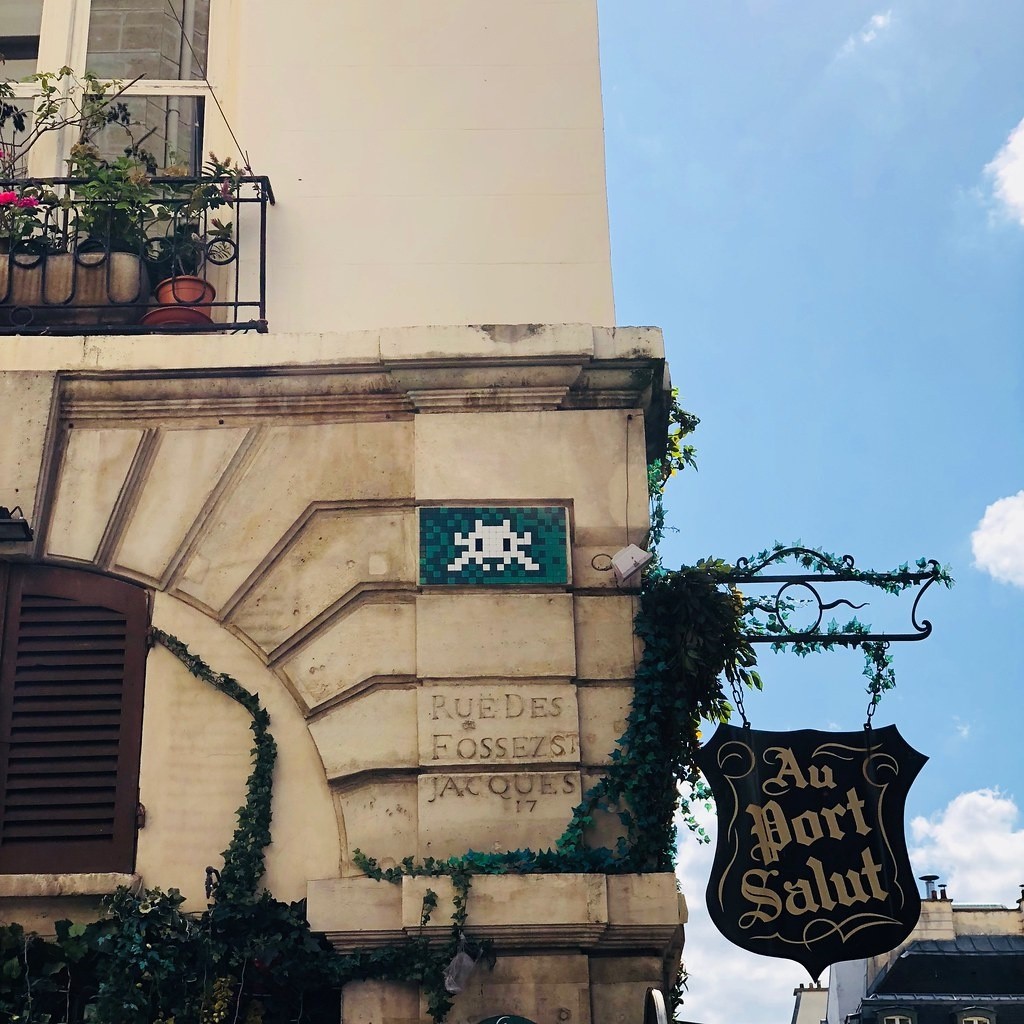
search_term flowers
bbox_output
[0,147,133,253]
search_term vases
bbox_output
[0,253,150,326]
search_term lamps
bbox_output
[611,543,655,582]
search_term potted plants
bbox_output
[147,232,215,315]
